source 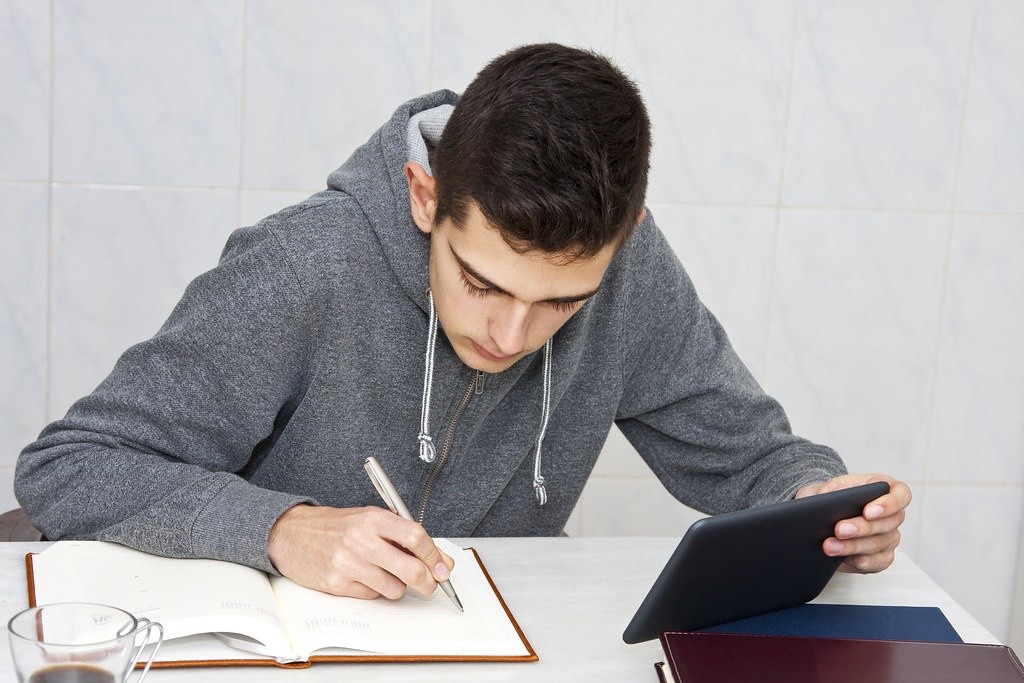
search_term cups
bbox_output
[7,602,137,683]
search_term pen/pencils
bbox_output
[359,455,471,617]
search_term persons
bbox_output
[15,42,912,599]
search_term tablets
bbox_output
[622,482,890,645]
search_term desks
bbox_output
[0,517,1016,683]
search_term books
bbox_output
[25,538,539,668]
[655,604,1024,683]
